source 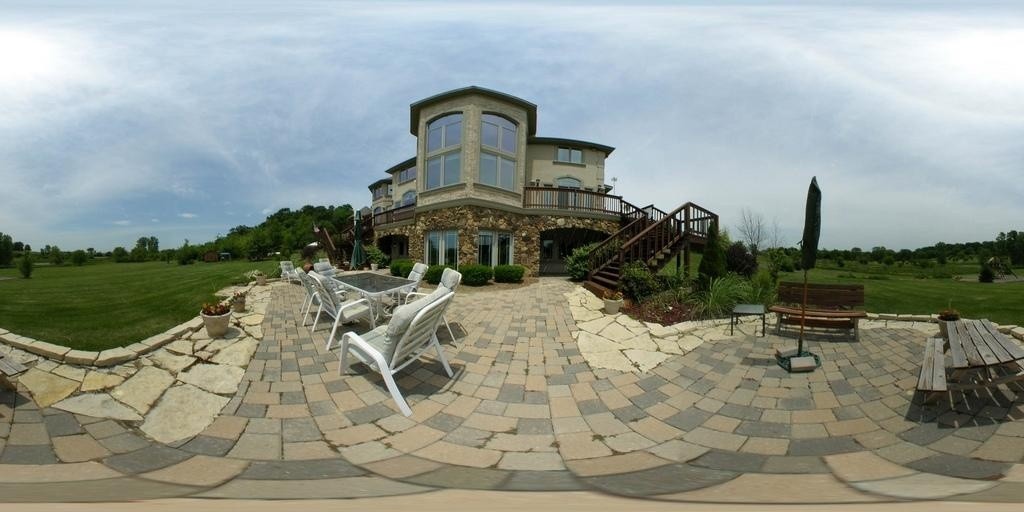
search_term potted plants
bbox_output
[939,309,960,337]
[367,245,383,271]
[256,273,267,285]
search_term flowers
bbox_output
[603,288,623,300]
[202,291,245,314]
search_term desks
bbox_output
[730,303,766,336]
[948,321,1023,402]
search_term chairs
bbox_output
[280,257,462,417]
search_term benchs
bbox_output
[769,281,865,343]
[918,338,1023,402]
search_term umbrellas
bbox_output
[348,210,362,271]
[795,176,822,356]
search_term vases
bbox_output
[603,297,623,314]
[200,310,232,338]
